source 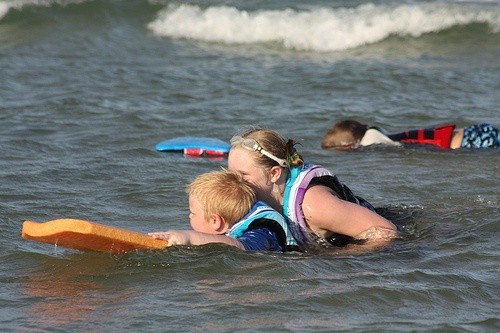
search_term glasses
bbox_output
[231,135,290,168]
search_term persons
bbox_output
[322,121,500,155]
[147,167,308,252]
[229,128,423,252]
